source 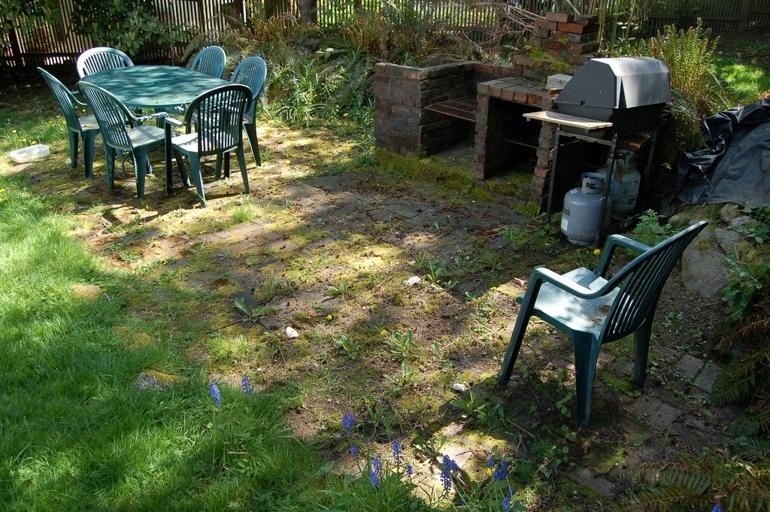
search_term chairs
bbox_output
[36,44,268,209]
[497,219,709,424]
